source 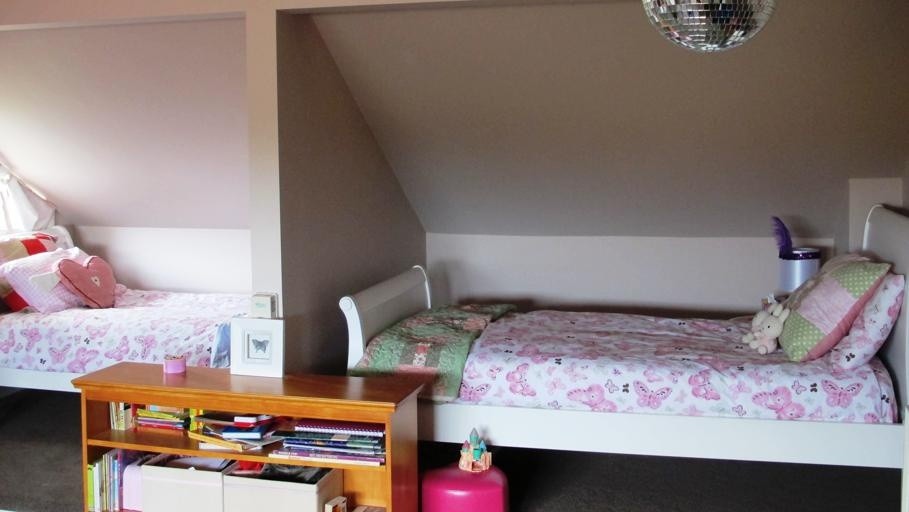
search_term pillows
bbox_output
[760,248,907,373]
[1,233,128,316]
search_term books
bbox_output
[81,401,385,512]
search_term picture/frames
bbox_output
[230,318,285,377]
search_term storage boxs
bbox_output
[141,455,343,512]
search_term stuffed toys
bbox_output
[749,303,791,356]
[741,300,781,346]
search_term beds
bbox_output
[337,200,909,512]
[0,226,253,395]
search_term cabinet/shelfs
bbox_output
[71,360,428,512]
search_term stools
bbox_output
[420,462,509,512]
